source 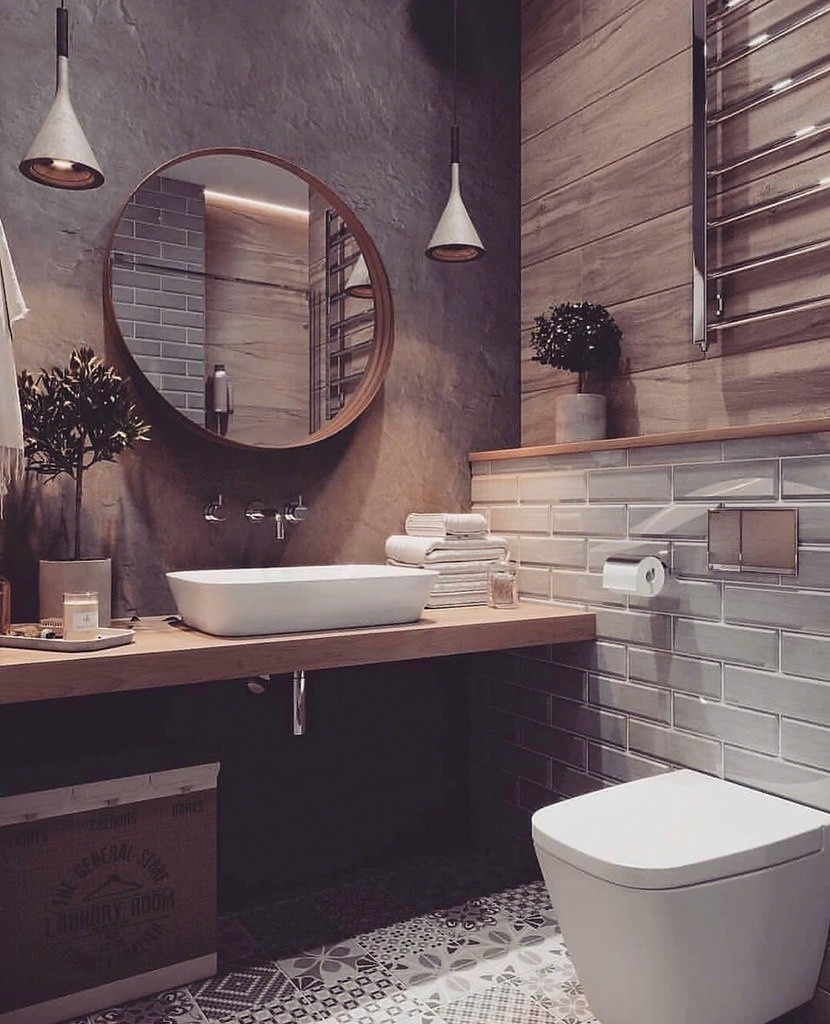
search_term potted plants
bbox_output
[15,341,154,627]
[528,301,624,444]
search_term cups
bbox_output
[485,560,520,610]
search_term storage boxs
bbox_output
[1,760,224,1024]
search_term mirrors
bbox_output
[102,144,396,456]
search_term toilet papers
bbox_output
[601,555,666,597]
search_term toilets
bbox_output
[532,768,830,1024]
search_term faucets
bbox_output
[244,493,286,541]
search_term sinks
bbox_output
[162,563,441,638]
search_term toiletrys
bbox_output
[61,590,100,642]
[486,552,520,610]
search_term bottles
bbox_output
[63,591,98,642]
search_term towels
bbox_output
[384,511,512,609]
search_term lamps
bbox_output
[424,0,486,264]
[344,251,375,300]
[18,0,107,192]
